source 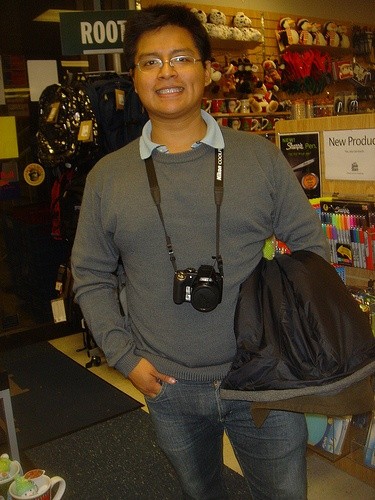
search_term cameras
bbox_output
[173,265,223,312]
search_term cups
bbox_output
[9,474,66,500]
[224,98,241,112]
[0,460,23,500]
[254,117,268,131]
[212,99,224,113]
[264,117,279,130]
[229,118,241,130]
[216,118,228,127]
[242,117,259,131]
[201,98,212,112]
[240,100,250,114]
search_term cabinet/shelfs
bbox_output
[210,111,291,136]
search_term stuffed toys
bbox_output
[190,7,264,42]
[211,57,281,113]
[280,17,350,47]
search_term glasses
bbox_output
[134,56,201,71]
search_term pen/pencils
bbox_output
[321,211,375,270]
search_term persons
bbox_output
[72,5,331,500]
